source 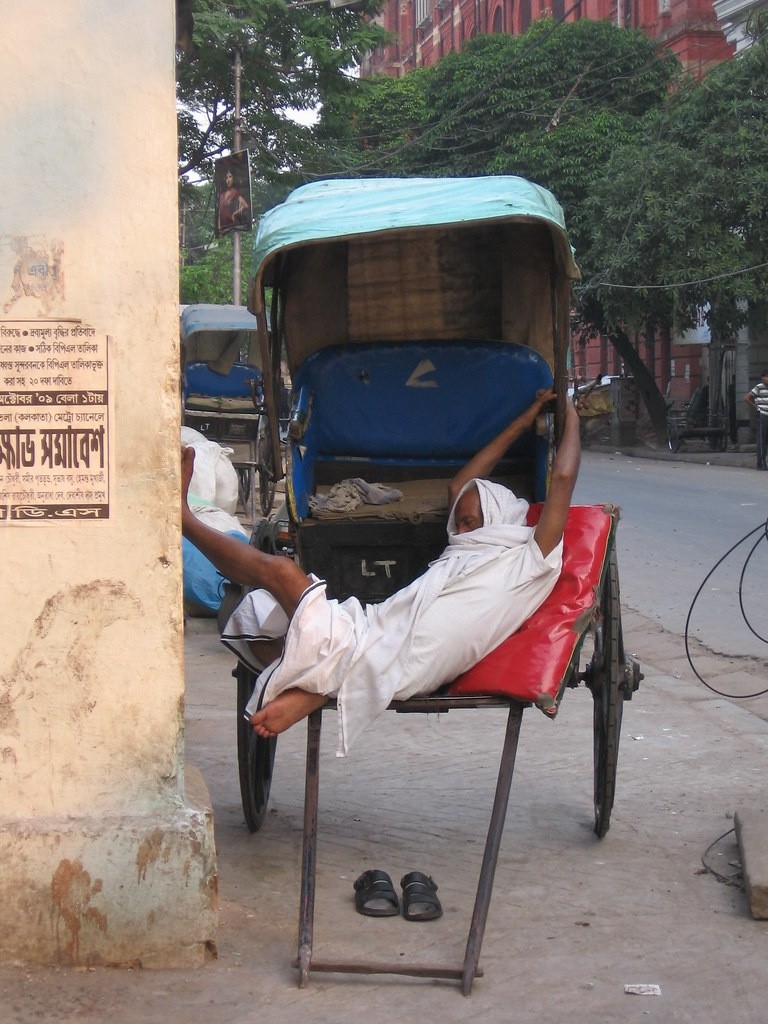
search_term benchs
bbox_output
[184,359,262,443]
[664,408,728,453]
[282,339,557,613]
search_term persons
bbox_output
[745,372,768,469]
[174,384,582,756]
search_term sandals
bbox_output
[353,869,399,916]
[401,872,444,921]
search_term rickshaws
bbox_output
[216,179,649,1007]
[178,303,285,510]
